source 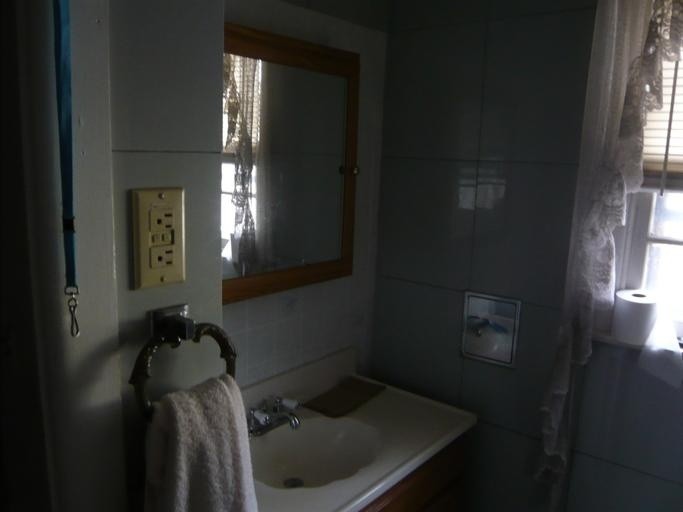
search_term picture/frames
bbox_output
[462,290,522,369]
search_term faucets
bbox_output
[266,394,303,431]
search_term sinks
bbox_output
[249,375,479,512]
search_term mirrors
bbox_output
[221,22,360,307]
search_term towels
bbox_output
[144,375,259,511]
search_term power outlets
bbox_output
[130,187,185,290]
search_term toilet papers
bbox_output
[611,289,657,348]
